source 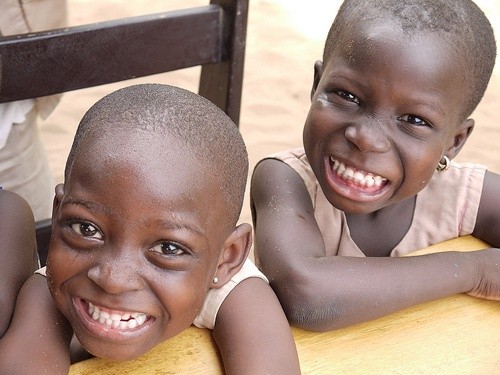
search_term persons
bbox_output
[0,84,302,375]
[0,189,42,338]
[0,0,69,222]
[248,0,500,332]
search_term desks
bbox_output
[70,229,500,375]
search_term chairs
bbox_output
[0,0,251,270]
